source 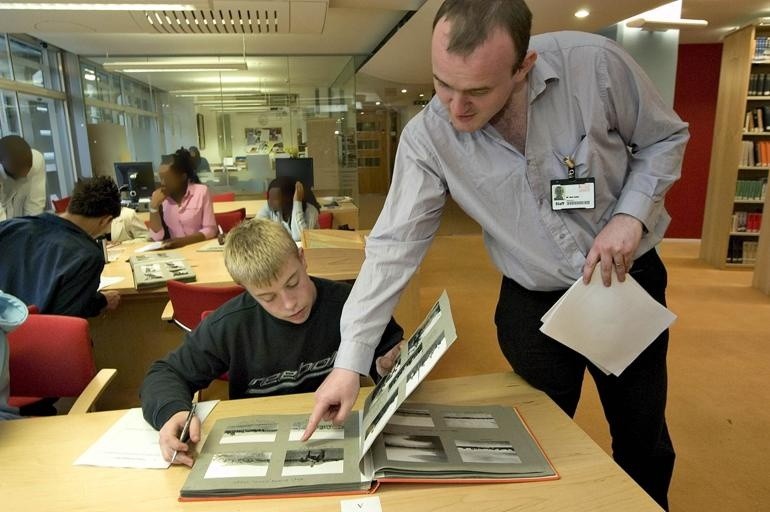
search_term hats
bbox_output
[0,135,32,177]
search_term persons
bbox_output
[138,218,405,468]
[0,289,30,419]
[100,207,145,240]
[554,186,565,199]
[0,134,46,224]
[256,176,320,241]
[149,145,220,247]
[301,0,690,512]
[0,173,126,317]
[189,146,211,172]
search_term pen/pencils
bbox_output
[217,223,225,244]
[171,389,201,464]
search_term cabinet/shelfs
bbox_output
[699,23,770,270]
[353,111,391,193]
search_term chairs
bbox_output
[0,193,331,417]
[208,154,248,172]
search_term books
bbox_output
[718,24,770,263]
[178,287,560,503]
[97,260,138,296]
[129,252,199,288]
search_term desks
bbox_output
[95,228,424,406]
[1,369,665,512]
[134,195,361,230]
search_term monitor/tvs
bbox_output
[276,157,314,190]
[114,162,156,203]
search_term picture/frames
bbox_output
[197,113,205,150]
